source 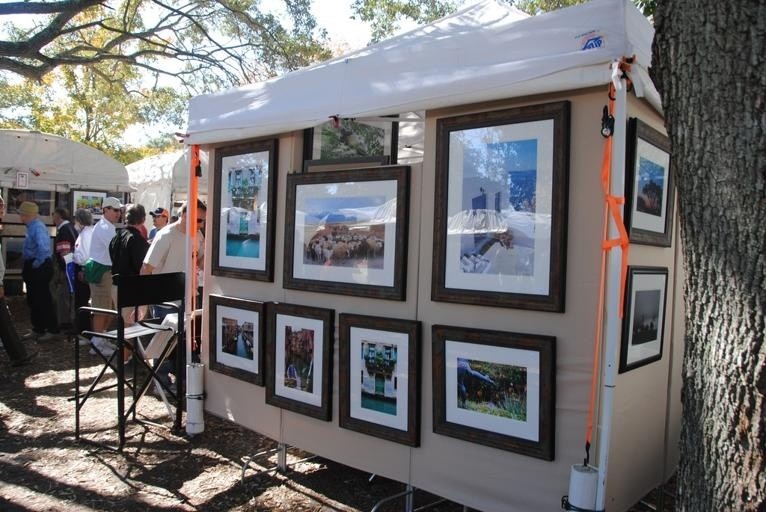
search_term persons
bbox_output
[15,202,59,343]
[88,197,126,355]
[52,209,78,328]
[130,199,206,397]
[71,207,95,346]
[0,197,41,368]
[149,208,169,237]
[110,204,150,365]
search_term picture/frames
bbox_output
[70,188,108,221]
[431,323,556,462]
[618,264,669,373]
[283,164,410,303]
[338,312,422,448]
[208,294,265,386]
[302,155,389,173]
[264,301,335,422]
[211,139,279,282]
[301,114,399,166]
[626,115,676,247]
[430,99,571,315]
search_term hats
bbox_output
[102,196,125,209]
[16,201,41,217]
[150,208,168,217]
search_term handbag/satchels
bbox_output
[62,252,75,294]
[83,258,112,283]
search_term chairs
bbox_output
[66,274,186,445]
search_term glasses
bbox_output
[197,218,204,223]
[113,209,121,212]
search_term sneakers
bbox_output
[37,332,54,342]
[103,339,119,351]
[20,331,38,339]
[11,349,41,366]
[89,344,114,357]
